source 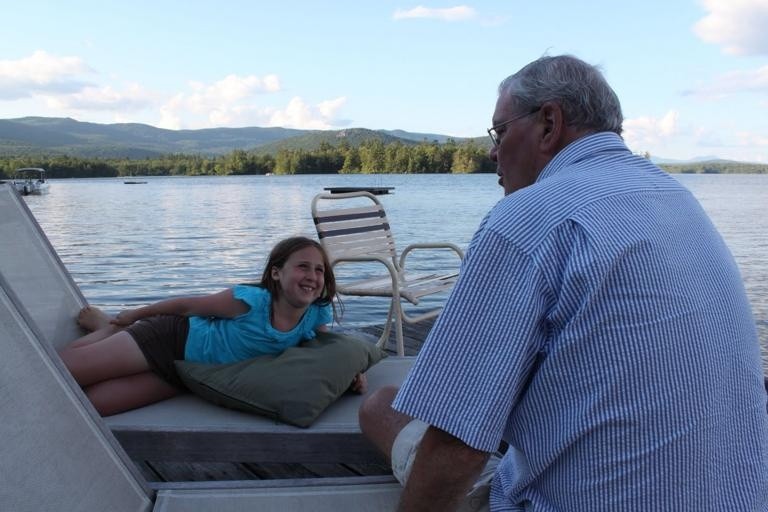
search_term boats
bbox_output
[11,167,50,197]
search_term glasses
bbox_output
[487,106,541,147]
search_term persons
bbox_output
[357,51,767,512]
[59,236,370,417]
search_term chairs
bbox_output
[311,190,465,356]
